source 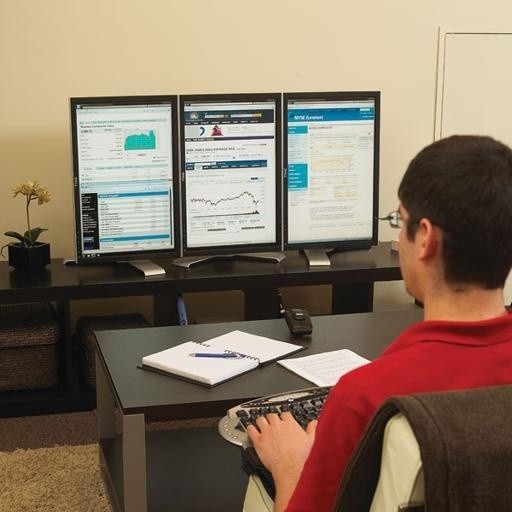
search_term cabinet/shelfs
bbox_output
[0,240,406,419]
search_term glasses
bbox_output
[390,210,423,230]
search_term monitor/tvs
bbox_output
[282,90,381,267]
[62,95,177,276]
[173,92,285,267]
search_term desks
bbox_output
[92,310,426,511]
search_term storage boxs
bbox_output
[0,303,60,392]
[78,312,152,392]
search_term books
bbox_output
[139,330,306,388]
[278,349,373,390]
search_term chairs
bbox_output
[242,386,512,512]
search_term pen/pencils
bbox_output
[190,352,240,358]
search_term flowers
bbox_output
[2,182,51,243]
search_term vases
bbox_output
[9,242,52,270]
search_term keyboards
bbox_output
[218,386,336,472]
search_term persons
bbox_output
[246,135,511,511]
